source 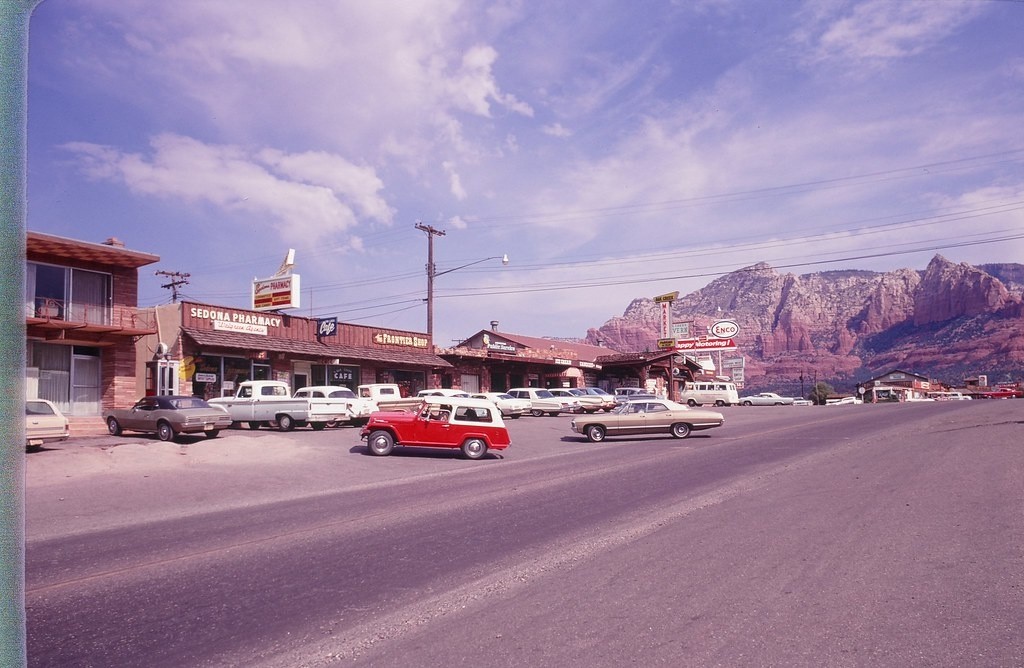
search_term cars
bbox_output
[25,399,70,452]
[578,387,667,412]
[507,388,581,417]
[358,396,512,460]
[981,387,1024,400]
[793,397,807,406]
[472,392,533,419]
[571,400,725,442]
[293,386,379,431]
[548,388,609,413]
[102,396,233,441]
[416,388,488,421]
[738,392,795,407]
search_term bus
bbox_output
[679,382,739,407]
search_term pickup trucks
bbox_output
[207,380,347,432]
[358,383,440,425]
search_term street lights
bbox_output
[427,253,508,354]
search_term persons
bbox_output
[636,403,645,413]
[428,404,449,422]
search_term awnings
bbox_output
[179,324,453,367]
